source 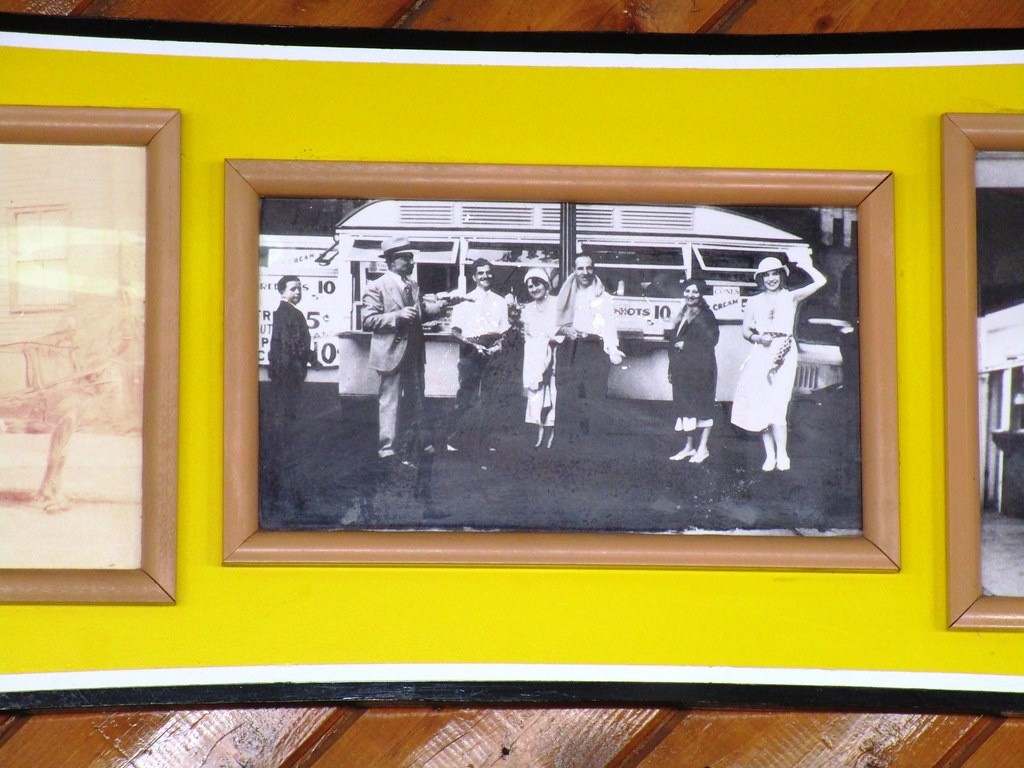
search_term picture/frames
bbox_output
[222,157,902,574]
[0,104,183,606]
[939,111,1024,633]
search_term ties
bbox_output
[389,280,412,350]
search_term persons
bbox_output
[520,268,558,449]
[448,257,512,453]
[668,278,719,463]
[267,275,311,466]
[730,249,827,472]
[361,235,475,476]
[549,252,626,457]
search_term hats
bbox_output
[377,234,420,258]
[524,267,552,291]
[753,257,790,280]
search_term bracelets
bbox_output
[749,334,755,340]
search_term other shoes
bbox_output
[777,454,791,471]
[381,452,401,466]
[690,450,710,463]
[669,447,696,460]
[762,454,778,472]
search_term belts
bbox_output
[576,332,603,344]
